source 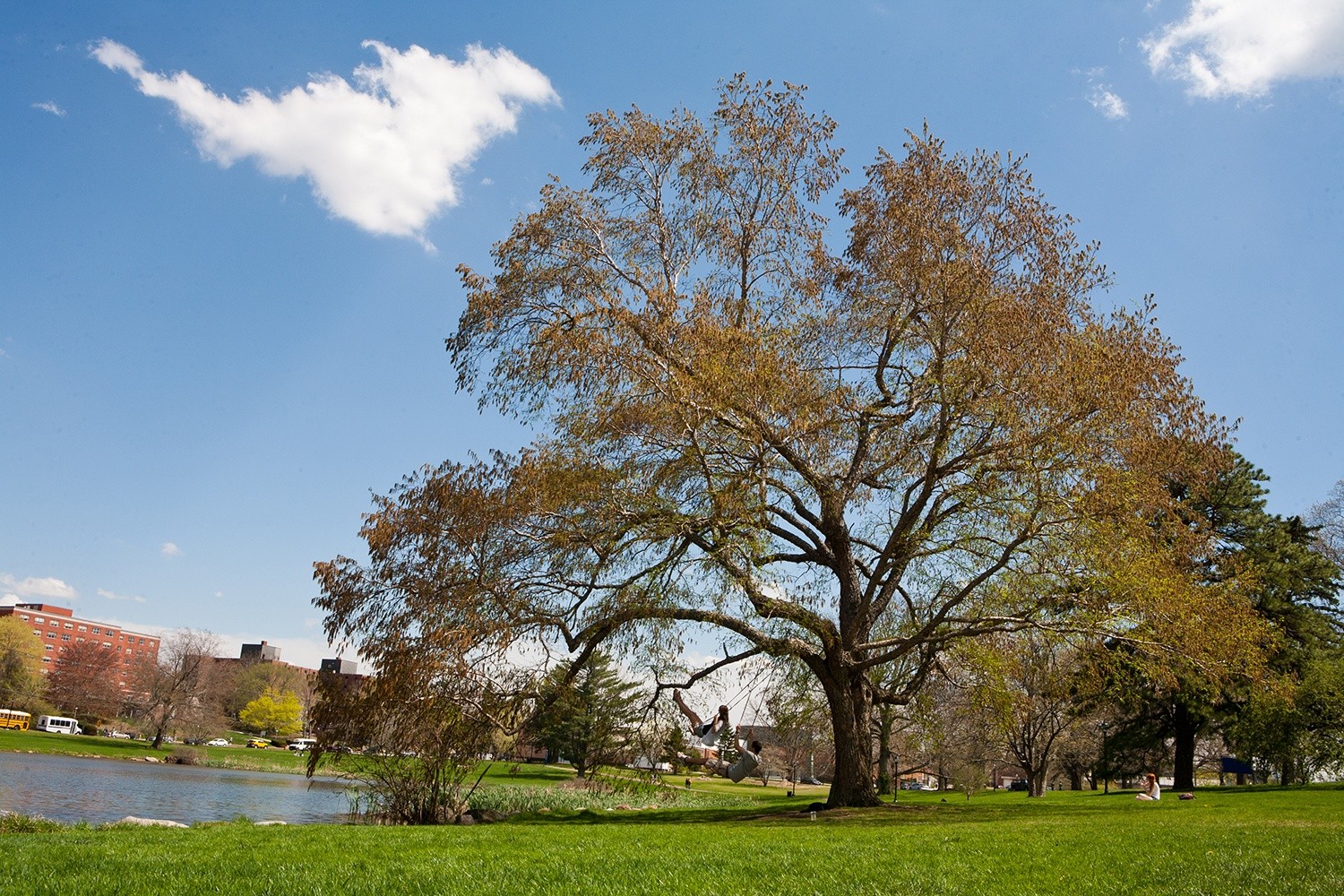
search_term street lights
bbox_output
[74,707,79,719]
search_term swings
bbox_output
[686,650,794,736]
[710,618,796,778]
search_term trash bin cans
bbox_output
[787,791,792,796]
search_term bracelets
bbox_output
[736,732,740,734]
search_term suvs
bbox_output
[247,739,269,750]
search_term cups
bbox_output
[810,811,816,821]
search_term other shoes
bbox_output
[677,751,688,767]
[673,689,681,701]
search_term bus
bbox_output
[0,709,32,732]
[36,715,82,735]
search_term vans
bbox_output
[288,738,317,751]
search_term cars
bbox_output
[328,744,494,761]
[108,730,130,740]
[901,782,930,790]
[208,738,228,747]
[184,736,208,745]
[149,735,174,743]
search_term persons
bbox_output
[673,689,728,745]
[1136,774,1160,802]
[676,724,762,783]
